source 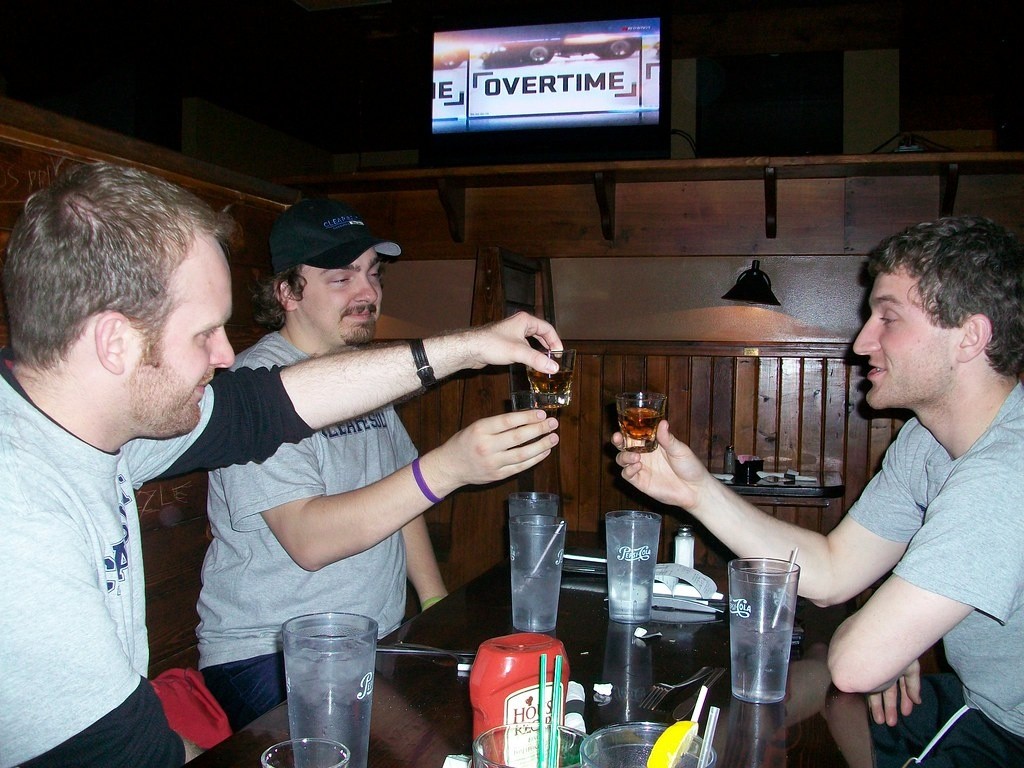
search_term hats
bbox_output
[269,198,402,275]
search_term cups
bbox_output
[509,514,566,633]
[526,348,576,408]
[473,723,599,768]
[281,612,378,768]
[579,720,718,768]
[260,736,351,768]
[605,510,662,624]
[508,492,559,518]
[511,391,558,446]
[602,621,652,701]
[729,558,801,705]
[616,392,668,453]
[735,459,764,484]
[724,699,787,768]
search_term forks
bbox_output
[638,667,714,711]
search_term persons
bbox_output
[610,215,1024,768]
[2,160,563,768]
[195,193,559,733]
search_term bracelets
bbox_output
[408,338,437,386]
[421,597,442,611]
[413,458,445,503]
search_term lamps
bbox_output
[722,259,782,307]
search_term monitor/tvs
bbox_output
[418,12,671,169]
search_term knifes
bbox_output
[673,668,727,720]
[374,645,476,655]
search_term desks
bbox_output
[710,472,846,534]
[179,544,877,768]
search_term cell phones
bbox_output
[564,544,606,562]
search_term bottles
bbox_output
[724,445,735,474]
[674,525,695,569]
[469,634,570,768]
